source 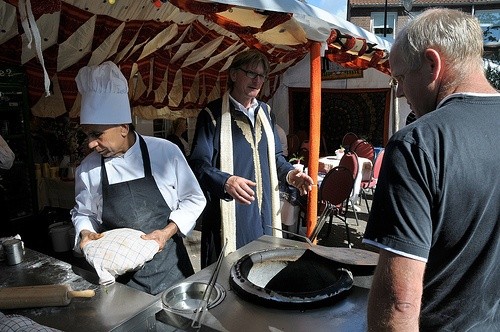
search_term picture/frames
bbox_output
[319,54,364,80]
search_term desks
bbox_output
[319,153,373,205]
[279,172,325,238]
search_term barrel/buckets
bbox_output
[69,228,86,258]
[48,222,73,252]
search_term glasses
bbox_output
[238,67,268,83]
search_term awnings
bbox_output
[0,0,395,243]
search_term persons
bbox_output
[362,7,500,332]
[0,131,15,170]
[69,60,207,297]
[187,49,315,271]
[166,117,190,158]
[273,123,288,160]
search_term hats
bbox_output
[75,61,133,125]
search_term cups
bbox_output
[1,239,24,266]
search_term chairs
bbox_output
[297,139,385,242]
